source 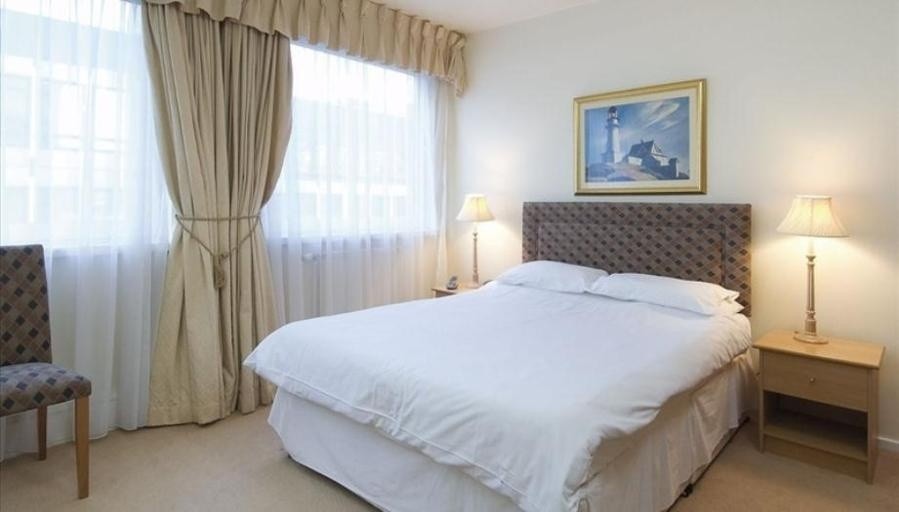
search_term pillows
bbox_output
[584,271,738,315]
[498,258,610,295]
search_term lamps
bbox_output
[777,194,854,344]
[454,191,496,289]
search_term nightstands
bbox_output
[751,325,888,486]
[428,278,493,297]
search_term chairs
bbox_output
[0,243,94,501]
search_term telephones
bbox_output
[447,275,459,289]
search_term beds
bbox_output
[271,201,757,512]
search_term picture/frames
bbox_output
[572,77,708,196]
